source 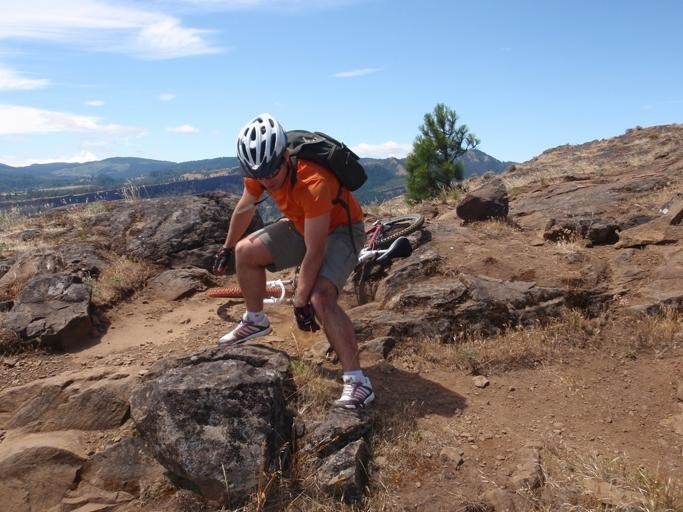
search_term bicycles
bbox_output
[207,213,425,307]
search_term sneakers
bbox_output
[219,314,273,347]
[334,375,375,410]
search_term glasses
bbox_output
[263,167,279,179]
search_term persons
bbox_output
[212,112,376,413]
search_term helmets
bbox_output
[235,112,287,179]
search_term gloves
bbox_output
[212,247,232,275]
[294,304,319,332]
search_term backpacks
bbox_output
[284,128,368,253]
